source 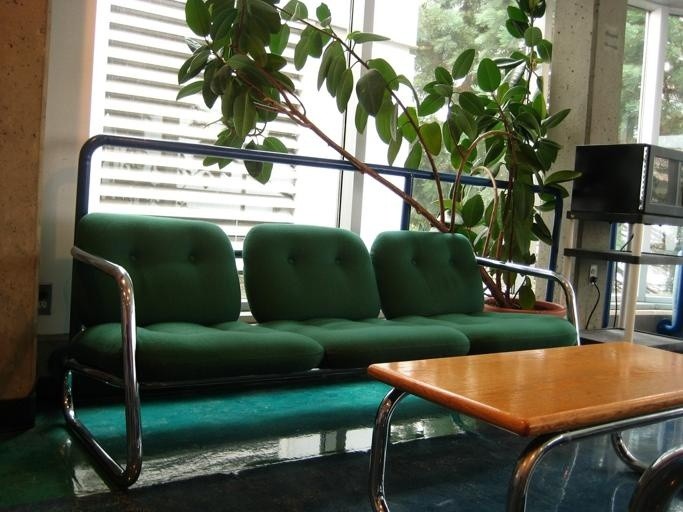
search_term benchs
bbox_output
[63,134,581,487]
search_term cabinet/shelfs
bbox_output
[565,204,681,352]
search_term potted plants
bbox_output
[174,0,587,322]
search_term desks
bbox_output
[364,337,683,511]
